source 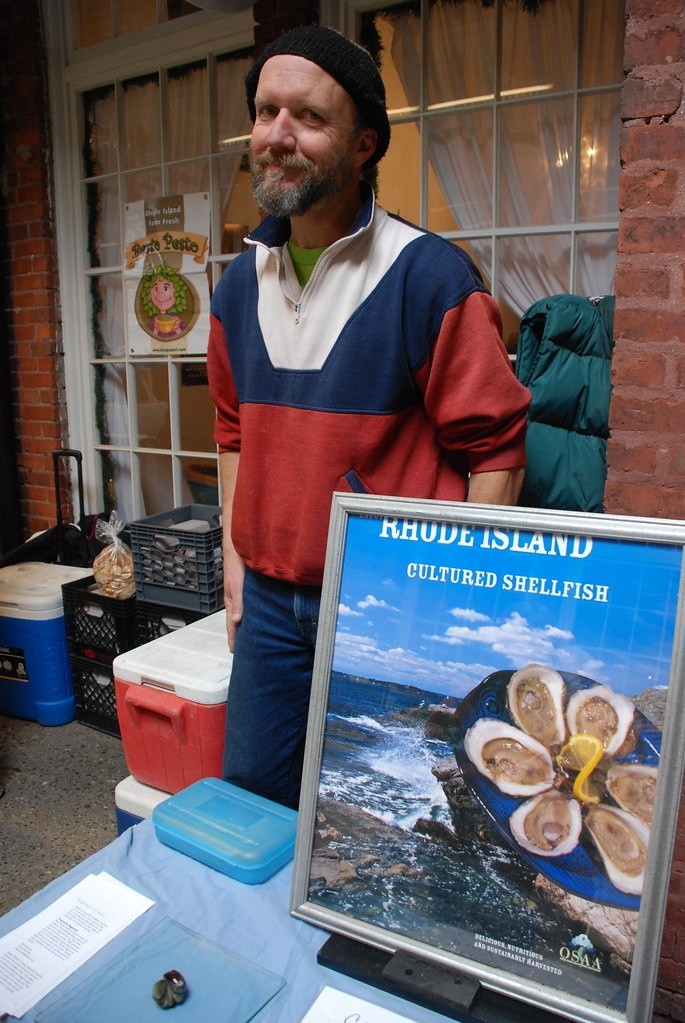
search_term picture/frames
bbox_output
[288,494,685,1023]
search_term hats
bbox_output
[242,25,392,173]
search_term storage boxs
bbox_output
[153,776,298,883]
[1,503,234,838]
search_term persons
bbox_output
[206,26,533,809]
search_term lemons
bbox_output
[556,735,605,803]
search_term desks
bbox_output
[1,822,576,1023]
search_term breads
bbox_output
[93,549,136,600]
